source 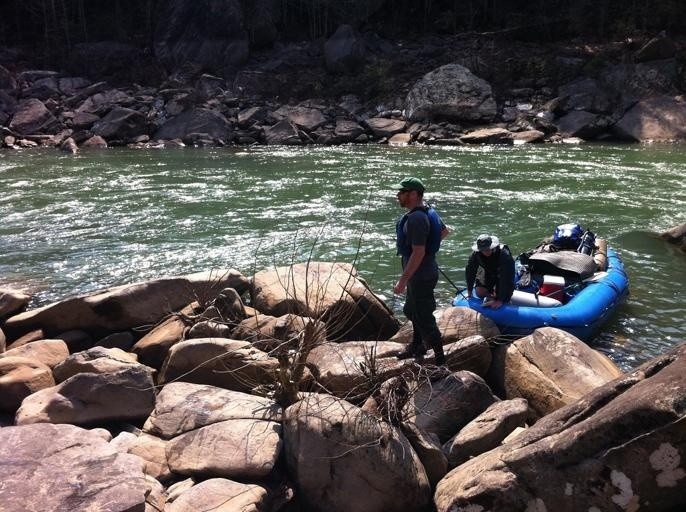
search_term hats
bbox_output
[389,176,426,192]
[471,233,500,252]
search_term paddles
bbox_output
[546,270,609,297]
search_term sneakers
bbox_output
[397,345,427,359]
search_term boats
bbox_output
[452,223,630,341]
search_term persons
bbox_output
[390,178,449,365]
[461,233,515,309]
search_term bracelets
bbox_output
[402,272,409,279]
